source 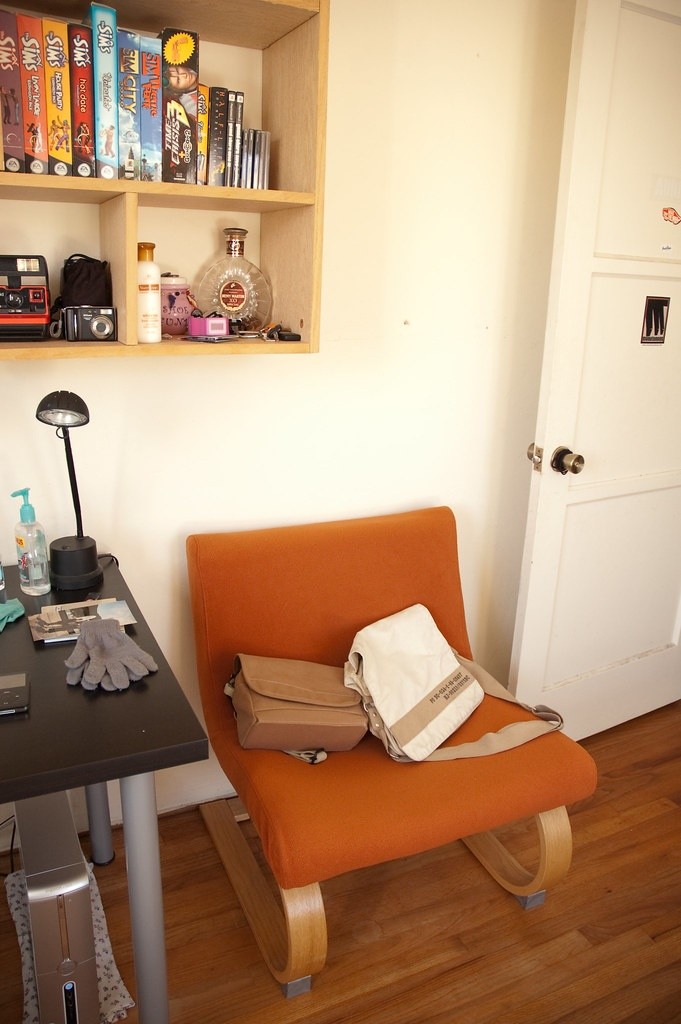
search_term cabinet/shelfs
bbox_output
[0,0,330,358]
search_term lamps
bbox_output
[35,390,104,589]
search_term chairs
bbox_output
[186,506,598,999]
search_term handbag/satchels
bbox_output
[62,254,113,309]
[225,650,370,765]
[343,605,563,763]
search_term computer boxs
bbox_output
[15,789,100,1024]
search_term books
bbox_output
[41,597,126,643]
[224,90,244,188]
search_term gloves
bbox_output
[65,620,160,691]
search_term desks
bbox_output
[0,553,210,1024]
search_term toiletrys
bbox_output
[136,241,161,344]
[10,488,52,597]
